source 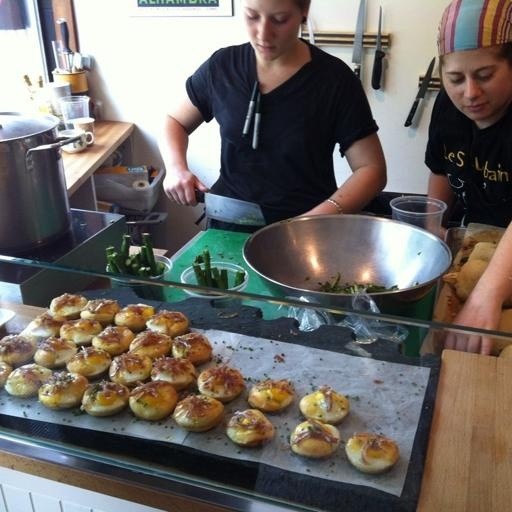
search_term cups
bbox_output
[389,196,448,235]
[40,70,96,132]
[104,252,174,300]
[181,261,250,303]
[56,129,95,154]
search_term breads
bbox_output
[299,385,349,424]
[345,433,400,474]
[196,365,245,404]
[247,379,293,413]
[224,409,275,449]
[173,395,225,433]
[290,420,340,460]
[0,292,213,420]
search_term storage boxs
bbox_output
[94,168,165,215]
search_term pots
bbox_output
[0,110,80,259]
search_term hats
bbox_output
[437,1,512,60]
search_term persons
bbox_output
[417,1,512,356]
[154,1,387,234]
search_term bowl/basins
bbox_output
[240,215,457,310]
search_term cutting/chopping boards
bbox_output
[433,222,512,352]
[416,347,511,511]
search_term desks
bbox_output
[62,120,135,211]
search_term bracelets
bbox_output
[326,198,345,215]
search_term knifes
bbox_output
[350,0,367,80]
[195,192,267,229]
[403,57,438,128]
